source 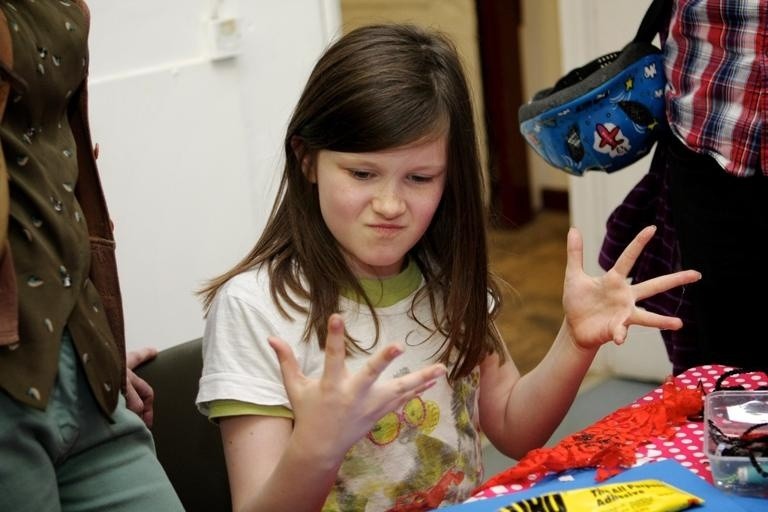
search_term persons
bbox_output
[1,0,187,512]
[591,0,766,378]
[190,21,704,512]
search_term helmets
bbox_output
[518,43,668,178]
